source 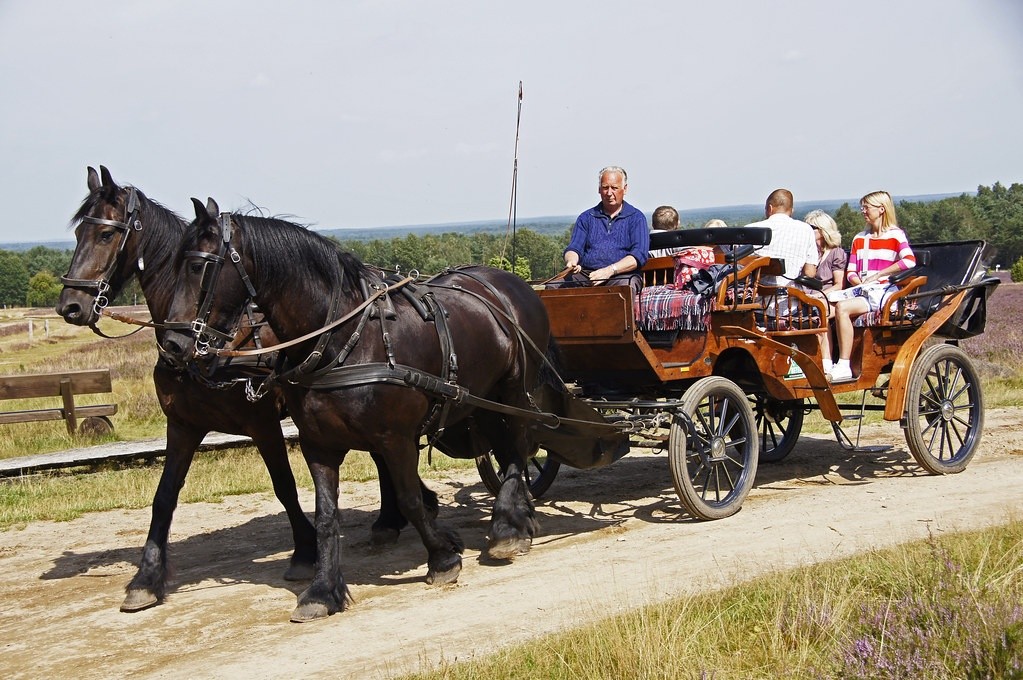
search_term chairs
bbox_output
[891,238,985,325]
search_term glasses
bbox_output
[810,225,819,230]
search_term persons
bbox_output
[556,165,650,298]
[741,189,820,329]
[701,219,729,252]
[814,191,916,384]
[647,205,681,257]
[802,209,847,299]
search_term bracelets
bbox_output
[610,264,617,276]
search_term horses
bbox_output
[56,165,568,624]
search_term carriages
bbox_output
[57,162,1003,630]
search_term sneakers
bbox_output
[827,363,853,381]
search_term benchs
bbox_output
[0,368,116,436]
